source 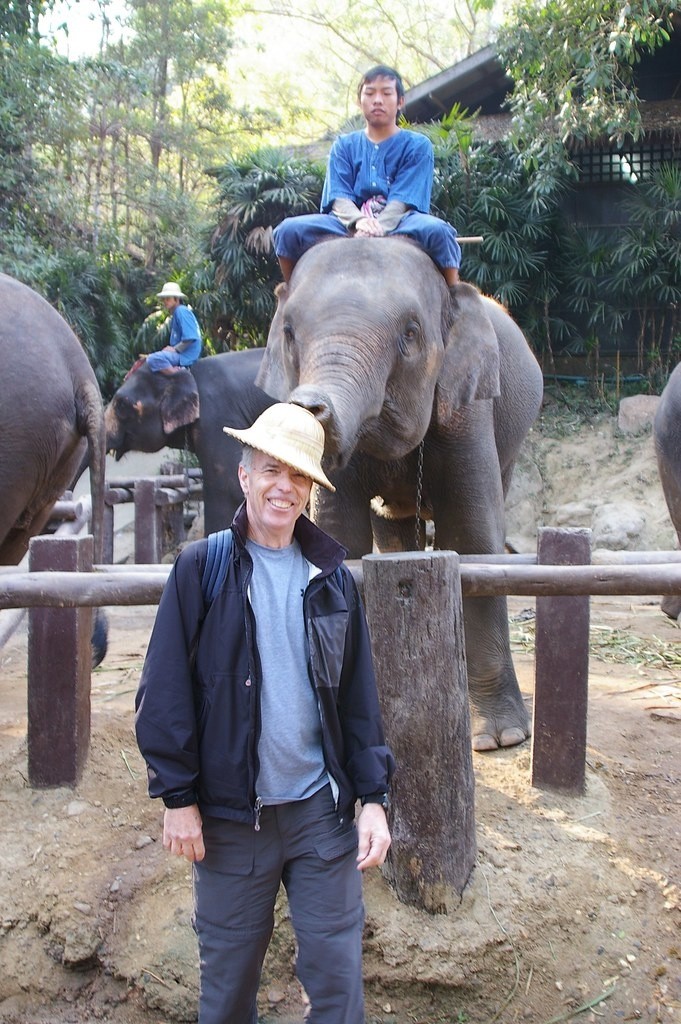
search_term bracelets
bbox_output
[359,794,390,811]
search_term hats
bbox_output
[221,401,338,494]
[157,282,188,299]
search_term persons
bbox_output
[272,66,463,283]
[135,401,395,1024]
[138,282,203,373]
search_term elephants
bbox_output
[65,347,283,553]
[252,233,543,751]
[1,273,109,670]
[653,361,681,620]
